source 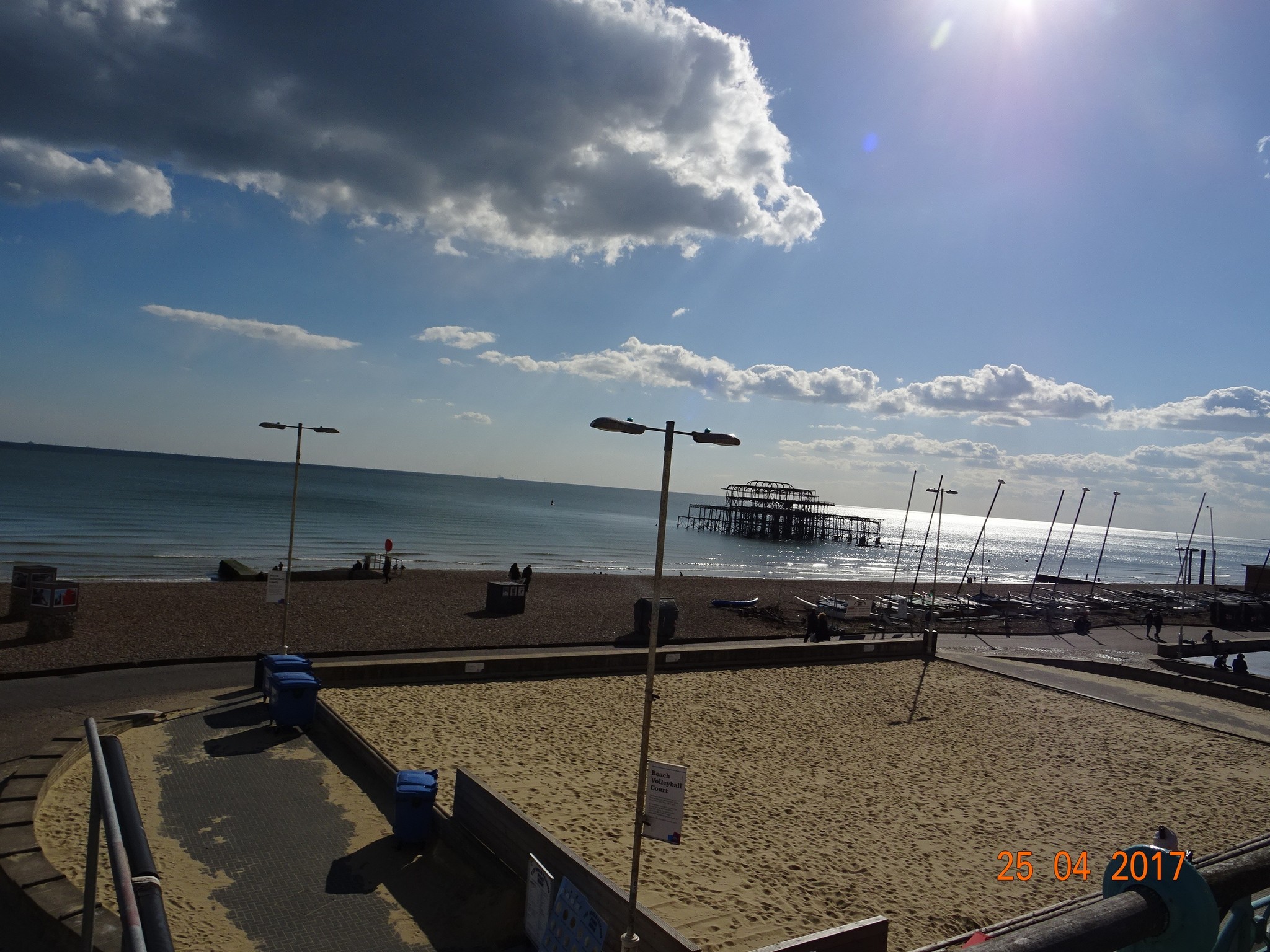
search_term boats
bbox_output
[712,597,758,607]
[944,587,1257,618]
[792,591,977,620]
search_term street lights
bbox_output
[596,414,740,952]
[259,422,340,657]
[1175,547,1199,658]
[926,488,959,656]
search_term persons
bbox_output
[353,560,369,570]
[1201,629,1219,654]
[1142,608,1163,638]
[924,606,937,629]
[1214,652,1232,671]
[984,576,988,583]
[974,576,976,581]
[508,563,533,591]
[278,562,283,571]
[33,590,48,605]
[272,566,278,570]
[1086,573,1088,580]
[1232,654,1249,674]
[804,608,828,643]
[680,572,683,576]
[382,556,391,584]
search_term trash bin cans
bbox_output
[261,654,313,710]
[267,670,322,734]
[486,581,526,616]
[254,652,279,691]
[634,597,679,636]
[394,768,438,851]
